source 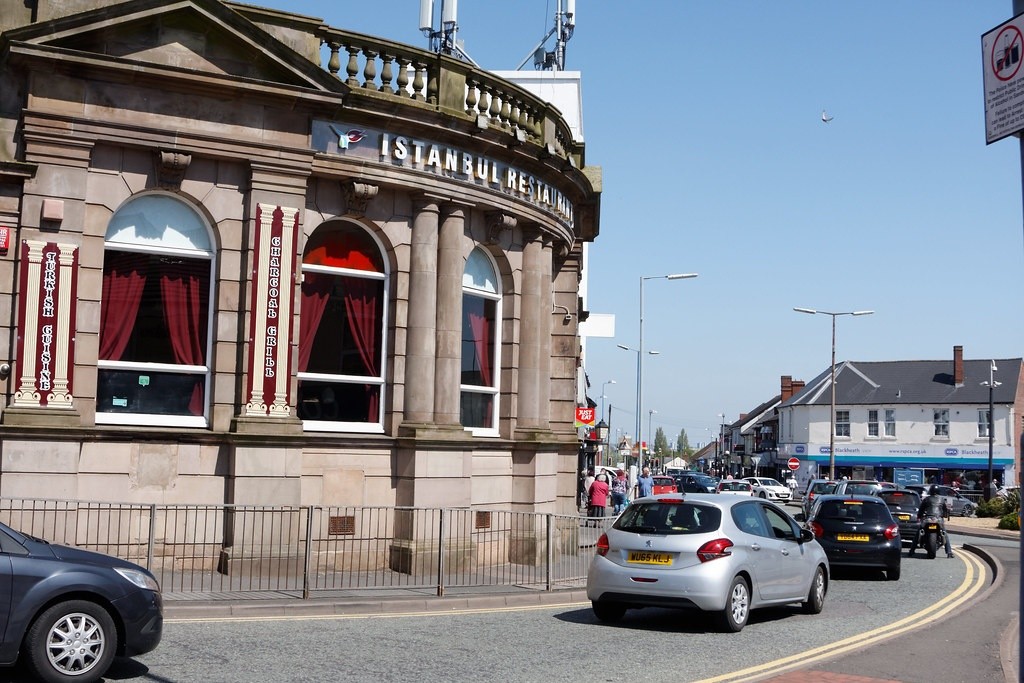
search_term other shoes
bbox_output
[947,553,954,558]
[593,521,603,527]
[909,549,914,554]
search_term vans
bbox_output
[583,465,623,480]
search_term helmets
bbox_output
[927,484,940,496]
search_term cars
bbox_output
[651,466,794,503]
[996,485,1020,501]
[798,478,977,520]
[0,522,163,683]
[803,495,902,581]
[586,492,830,634]
[878,486,922,543]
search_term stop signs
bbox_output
[788,458,800,470]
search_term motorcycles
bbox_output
[914,507,950,559]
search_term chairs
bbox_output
[820,503,840,517]
[640,510,666,531]
[863,505,880,520]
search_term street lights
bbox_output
[793,307,875,485]
[600,380,617,422]
[983,359,999,503]
[704,428,712,460]
[648,409,660,468]
[616,272,699,497]
[717,412,725,457]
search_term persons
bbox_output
[581,469,595,507]
[636,466,654,497]
[612,470,628,519]
[951,475,984,493]
[595,468,609,486]
[788,476,798,499]
[709,467,715,476]
[589,475,610,526]
[810,475,816,479]
[872,474,886,482]
[928,474,938,483]
[825,473,829,479]
[993,479,997,489]
[838,472,852,480]
[727,472,739,480]
[909,485,955,557]
